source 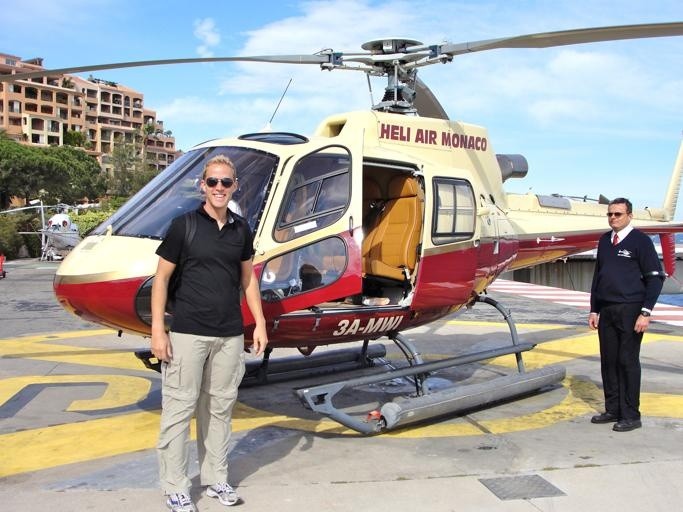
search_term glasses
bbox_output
[608,212,622,216]
[207,177,233,188]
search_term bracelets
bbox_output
[640,311,650,316]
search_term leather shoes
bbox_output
[593,409,620,423]
[612,421,644,432]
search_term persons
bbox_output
[148,155,268,512]
[587,198,666,432]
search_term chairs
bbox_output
[253,171,421,281]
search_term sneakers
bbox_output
[206,482,242,506]
[166,493,197,511]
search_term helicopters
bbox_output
[0,19,682,442]
[0,202,103,262]
